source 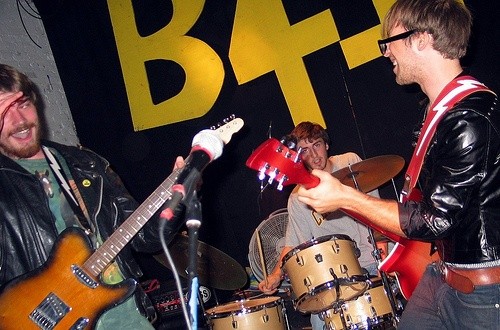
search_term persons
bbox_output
[0,64,203,330]
[259,122,396,330]
[298,0,500,330]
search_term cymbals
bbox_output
[331,154,406,194]
[153,232,249,291]
[368,231,391,244]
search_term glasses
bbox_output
[378,29,417,55]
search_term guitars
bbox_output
[245,135,441,302]
[0,112,245,330]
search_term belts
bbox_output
[432,257,500,293]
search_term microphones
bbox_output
[159,129,224,231]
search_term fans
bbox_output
[248,208,290,293]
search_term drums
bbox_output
[320,277,403,330]
[203,296,288,330]
[281,233,368,314]
